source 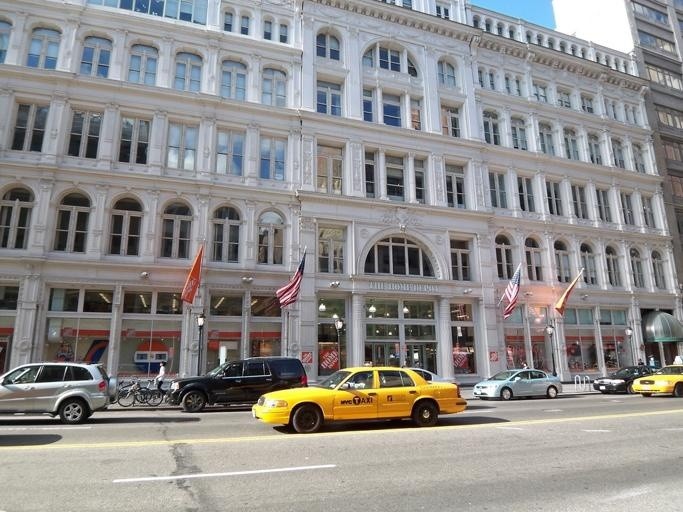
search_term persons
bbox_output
[154,361,166,398]
[330,374,368,391]
[638,359,644,375]
[522,363,529,369]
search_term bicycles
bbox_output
[110,379,163,407]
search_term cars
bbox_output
[473,369,562,400]
[593,365,683,398]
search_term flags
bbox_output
[555,271,582,315]
[181,245,203,304]
[275,250,307,309]
[501,263,521,319]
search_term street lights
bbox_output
[334,316,343,370]
[626,327,635,364]
[196,313,206,376]
[546,325,555,370]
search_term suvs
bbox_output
[0,363,117,424]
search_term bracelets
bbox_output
[348,384,350,388]
